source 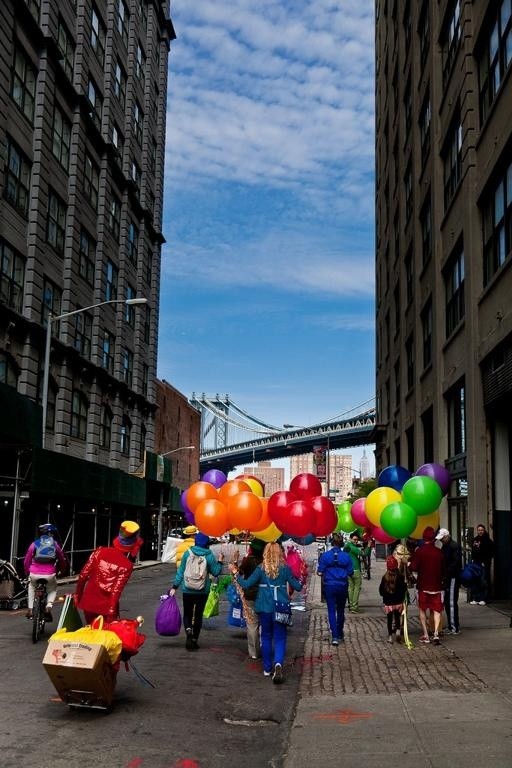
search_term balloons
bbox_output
[181,462,451,546]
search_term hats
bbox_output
[387,555,397,571]
[183,526,199,535]
[423,526,435,542]
[195,533,209,546]
[435,528,449,540]
[114,521,143,557]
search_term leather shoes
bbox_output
[44,609,52,622]
[25,609,33,617]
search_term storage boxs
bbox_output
[42,638,118,708]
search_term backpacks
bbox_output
[33,535,56,562]
[184,549,207,590]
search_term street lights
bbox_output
[157,445,196,561]
[336,465,361,487]
[41,298,149,450]
[283,424,329,568]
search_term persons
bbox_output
[23,523,66,622]
[469,525,494,606]
[317,534,372,646]
[315,450,325,465]
[170,526,223,650]
[73,520,143,683]
[229,538,307,683]
[380,526,461,645]
[317,464,326,476]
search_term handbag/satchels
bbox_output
[49,614,122,664]
[274,600,292,625]
[461,563,487,592]
[0,580,14,599]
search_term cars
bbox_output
[167,526,252,545]
[315,530,363,547]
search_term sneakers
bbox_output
[185,629,461,683]
[478,601,486,605]
[470,600,478,605]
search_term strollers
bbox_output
[0,560,39,611]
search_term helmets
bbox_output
[38,524,58,534]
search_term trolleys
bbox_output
[64,685,111,715]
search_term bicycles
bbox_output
[26,566,70,644]
[357,554,370,580]
